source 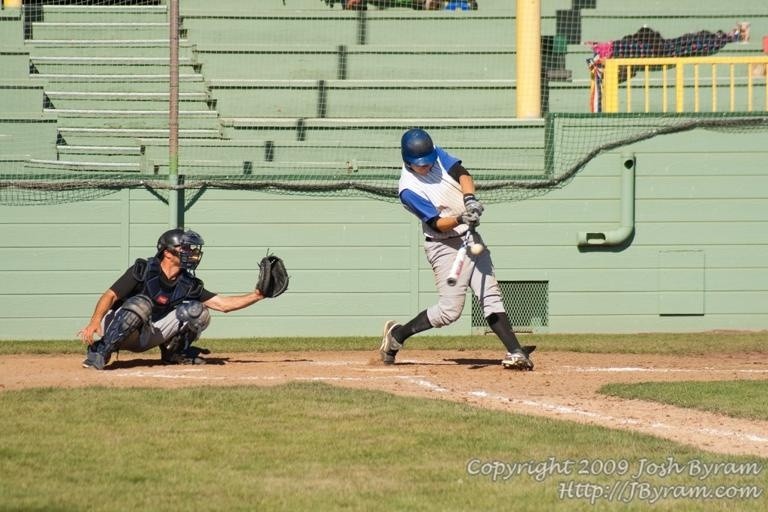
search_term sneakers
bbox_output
[378,319,400,365]
[502,349,534,371]
[82,359,95,368]
[162,354,207,364]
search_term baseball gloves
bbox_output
[257,254,289,297]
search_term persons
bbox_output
[74,228,289,371]
[378,129,533,369]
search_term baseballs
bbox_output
[471,243,484,256]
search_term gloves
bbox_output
[457,212,480,226]
[462,193,484,215]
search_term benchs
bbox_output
[0,10,58,163]
[136,0,768,177]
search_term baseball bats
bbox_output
[447,213,478,287]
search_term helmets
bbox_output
[401,128,435,167]
[156,230,204,271]
